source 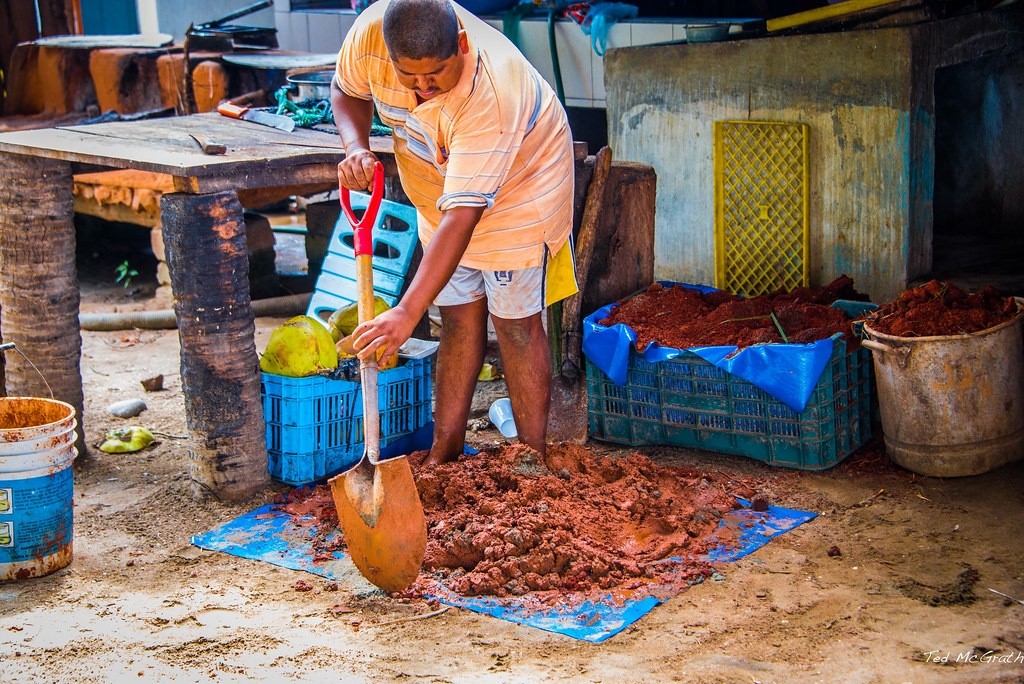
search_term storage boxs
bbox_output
[256,349,435,485]
[581,279,883,470]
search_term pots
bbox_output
[187,21,278,50]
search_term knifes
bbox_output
[216,101,296,133]
[187,130,227,155]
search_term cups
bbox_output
[489,398,519,438]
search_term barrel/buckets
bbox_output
[861,296,1024,477]
[0,342,79,583]
[682,22,731,44]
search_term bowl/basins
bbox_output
[287,70,338,104]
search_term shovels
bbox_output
[328,162,427,594]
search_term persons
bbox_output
[327,0,575,474]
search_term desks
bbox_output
[1,106,434,502]
